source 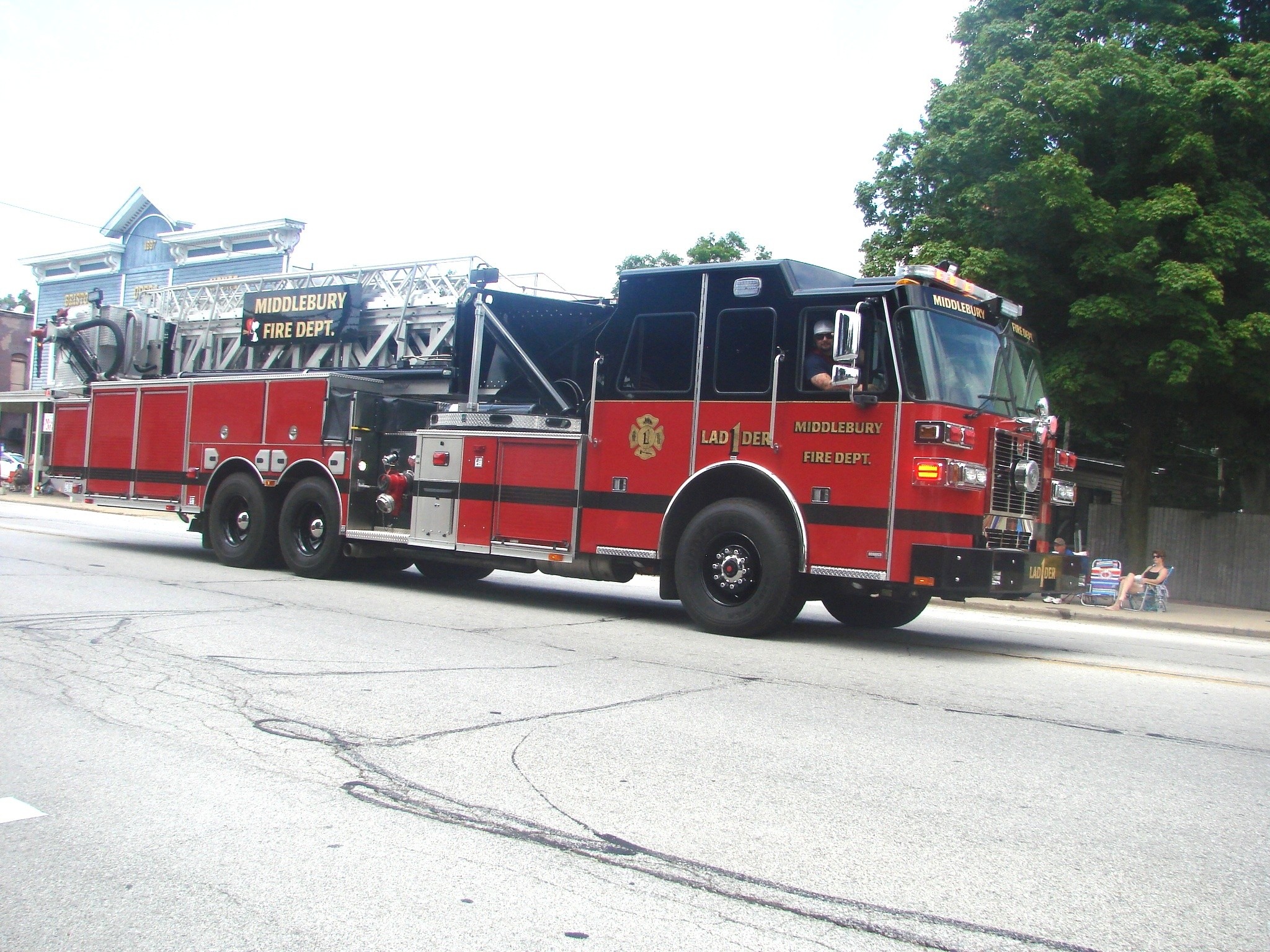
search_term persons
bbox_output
[1043,538,1075,604]
[1104,550,1168,610]
[23,449,44,489]
[12,464,29,492]
[803,319,880,393]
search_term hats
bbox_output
[1052,538,1065,545]
[17,464,22,468]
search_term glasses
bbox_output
[1153,555,1161,559]
[814,333,834,340]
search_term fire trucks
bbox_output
[31,258,1080,635]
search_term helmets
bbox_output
[813,319,835,334]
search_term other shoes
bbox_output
[12,490,20,492]
[1105,605,1120,611]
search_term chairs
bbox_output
[4,471,32,491]
[1080,558,1121,608]
[1121,566,1173,611]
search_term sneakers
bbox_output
[1042,595,1055,603]
[1052,598,1062,605]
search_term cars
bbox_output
[0,449,26,480]
